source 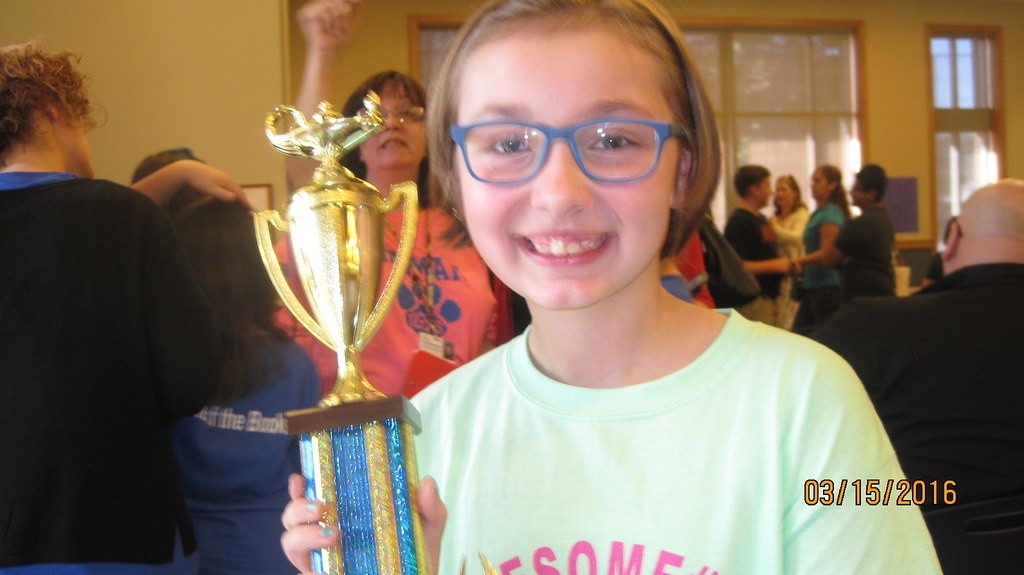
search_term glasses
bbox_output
[352,102,424,126]
[449,119,685,187]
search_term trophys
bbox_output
[251,89,436,574]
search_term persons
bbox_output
[1,45,322,574]
[725,165,895,334]
[280,0,943,574]
[809,178,1024,575]
[921,253,944,286]
[275,0,498,399]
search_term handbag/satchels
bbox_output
[696,211,760,308]
